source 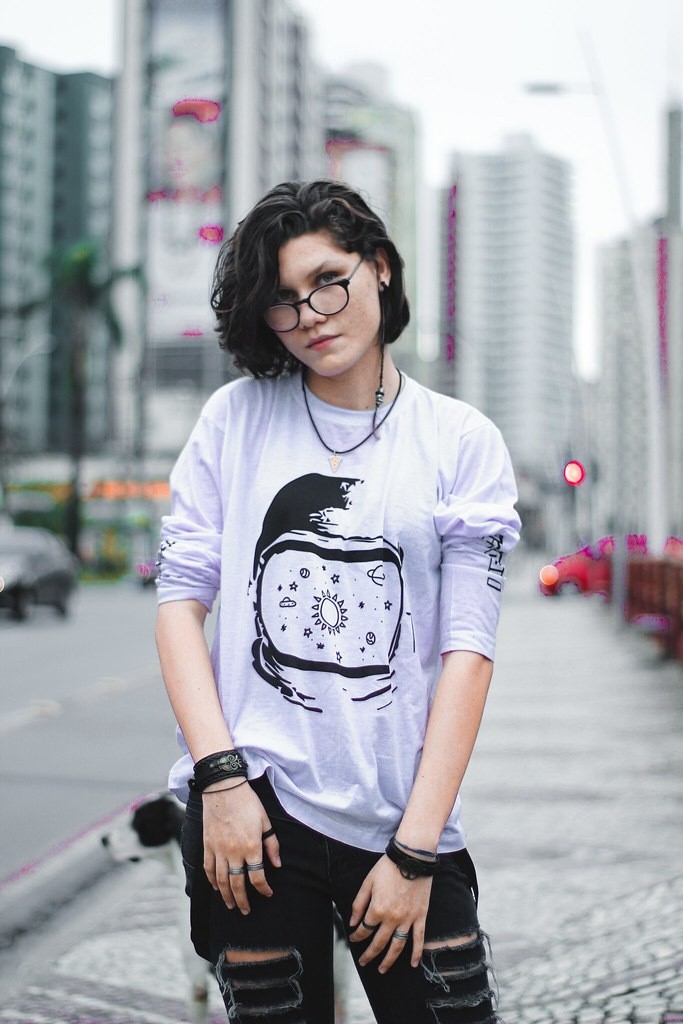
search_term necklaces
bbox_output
[299,363,403,474]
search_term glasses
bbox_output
[262,254,366,333]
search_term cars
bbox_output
[1,526,78,623]
[536,535,681,603]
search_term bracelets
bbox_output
[192,746,249,796]
[385,839,441,881]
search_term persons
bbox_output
[152,175,523,1022]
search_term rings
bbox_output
[362,917,376,932]
[247,862,264,872]
[393,929,408,942]
[228,866,245,875]
[260,827,276,841]
